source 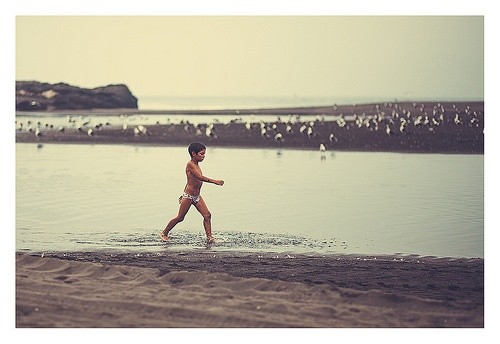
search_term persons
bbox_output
[158,142,225,243]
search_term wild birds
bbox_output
[17,99,484,151]
